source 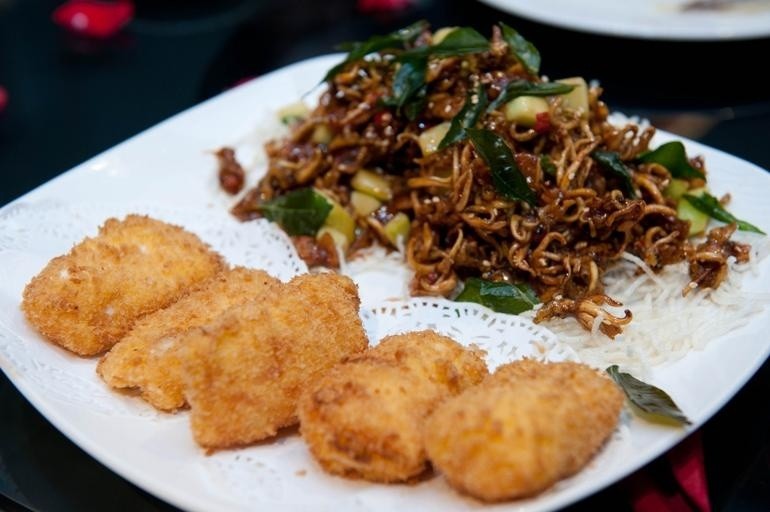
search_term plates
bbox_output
[0,53,768,512]
[479,0,769,42]
